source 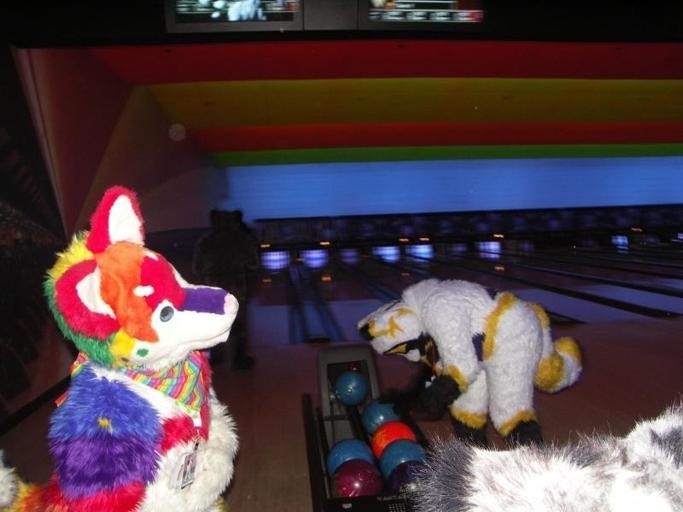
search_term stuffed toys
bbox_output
[356,274,585,448]
[405,401,683,512]
[1,184,242,511]
